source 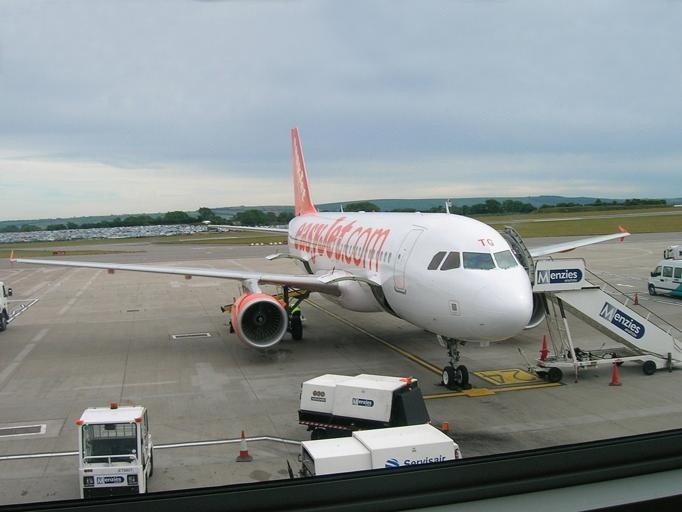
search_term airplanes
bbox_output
[5,126,630,391]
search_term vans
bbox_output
[646,259,682,299]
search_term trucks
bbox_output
[0,281,13,330]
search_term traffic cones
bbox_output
[609,365,621,386]
[539,334,549,359]
[633,293,639,305]
[235,429,253,462]
